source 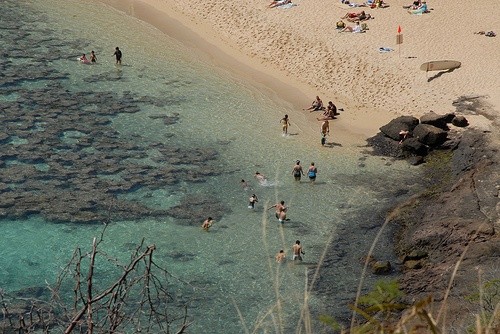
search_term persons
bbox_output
[268,0,292,8]
[266,200,287,220]
[80,54,88,63]
[398,129,409,145]
[240,179,252,191]
[248,194,258,209]
[202,217,214,232]
[275,250,286,263]
[403,0,430,15]
[338,0,390,33]
[291,160,305,183]
[280,114,291,135]
[306,162,318,183]
[253,170,268,182]
[279,207,287,224]
[90,50,97,63]
[302,96,337,146]
[292,239,305,265]
[112,47,122,65]
